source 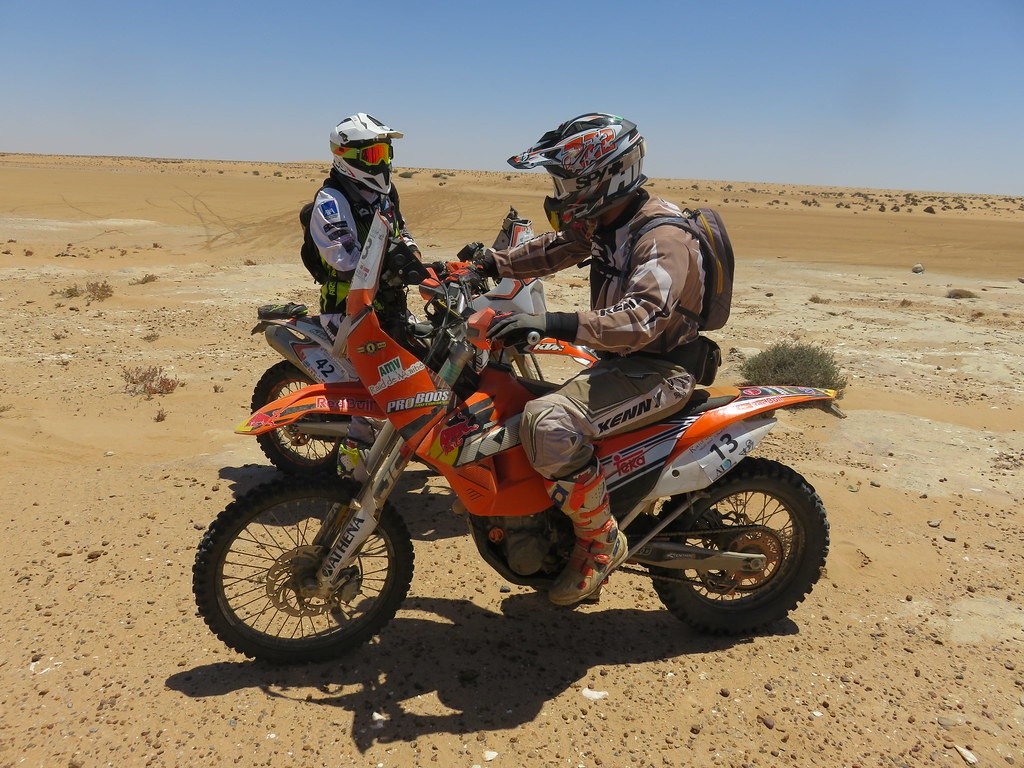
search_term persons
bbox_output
[300,112,423,482]
[469,109,708,610]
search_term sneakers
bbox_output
[334,436,370,489]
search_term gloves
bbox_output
[485,309,547,349]
[468,255,499,281]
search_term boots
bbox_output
[541,453,629,606]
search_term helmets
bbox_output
[505,110,649,234]
[328,113,406,195]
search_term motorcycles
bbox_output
[249,206,600,484]
[190,259,846,669]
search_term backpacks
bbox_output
[619,206,735,331]
[299,182,360,286]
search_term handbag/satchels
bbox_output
[695,335,722,386]
